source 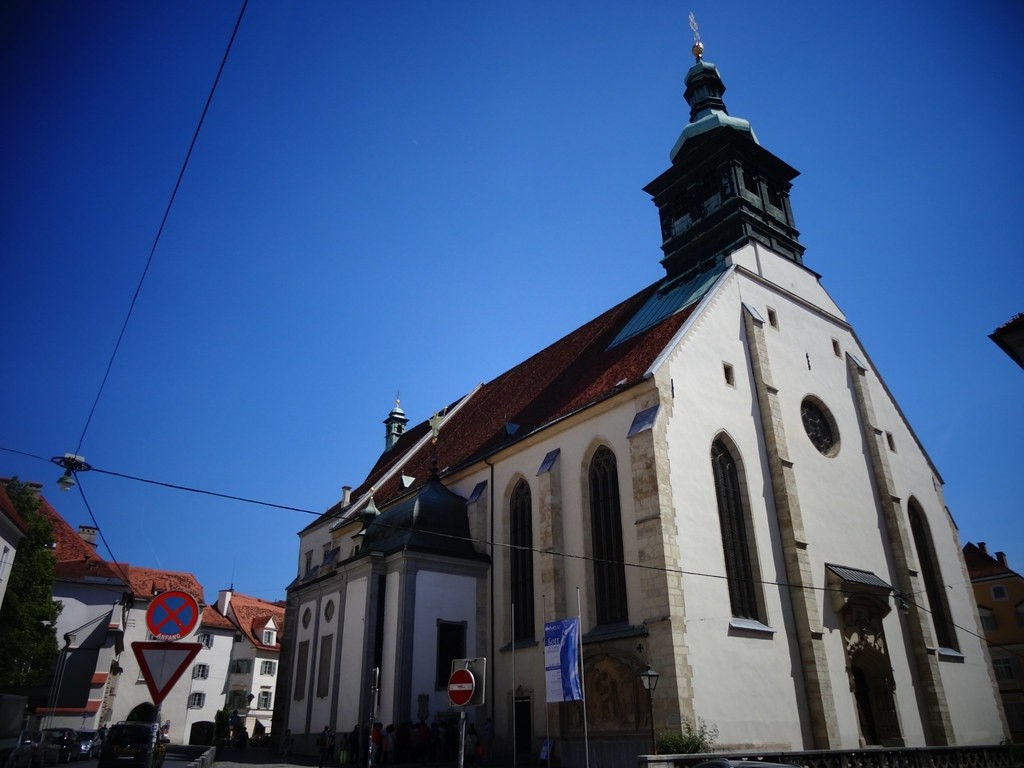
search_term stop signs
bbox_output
[448,669,475,705]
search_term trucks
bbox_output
[97,720,170,768]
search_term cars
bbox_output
[0,727,36,768]
[31,726,110,768]
[248,733,272,748]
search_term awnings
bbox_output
[825,563,896,591]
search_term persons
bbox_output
[234,724,248,753]
[315,715,495,766]
[280,729,293,763]
